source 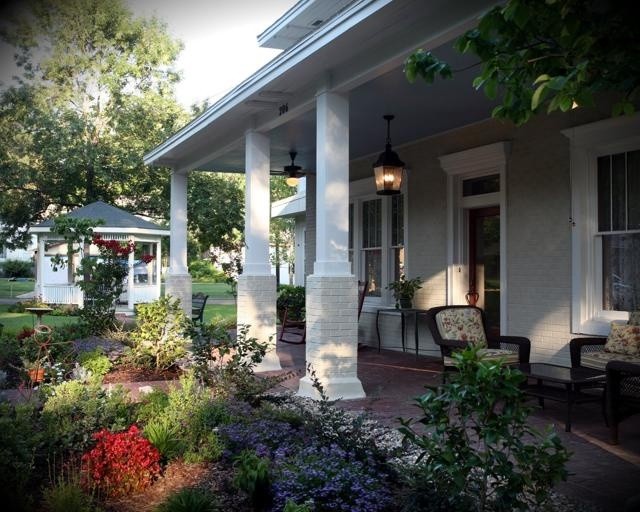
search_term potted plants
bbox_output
[23,331,63,382]
[385,276,423,308]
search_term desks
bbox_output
[507,360,607,431]
[375,307,431,358]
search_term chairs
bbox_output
[428,304,532,424]
[279,280,368,347]
[569,306,639,429]
[192,293,208,324]
